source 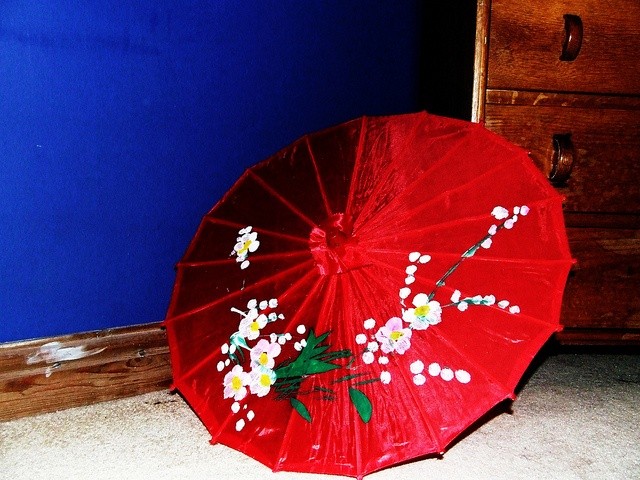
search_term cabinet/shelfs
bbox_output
[419,1,640,352]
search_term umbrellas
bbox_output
[159,110,578,479]
